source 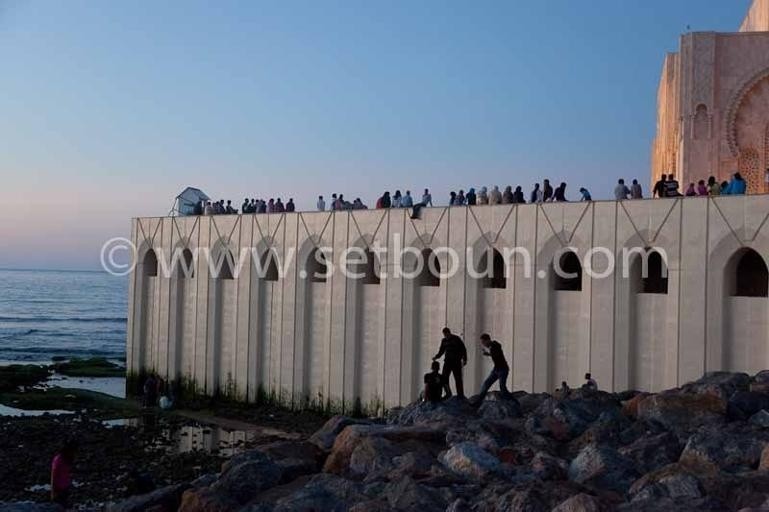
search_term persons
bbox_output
[433,329,467,400]
[420,362,451,402]
[555,374,597,391]
[50,444,74,509]
[184,172,747,216]
[469,334,520,408]
[136,369,175,411]
